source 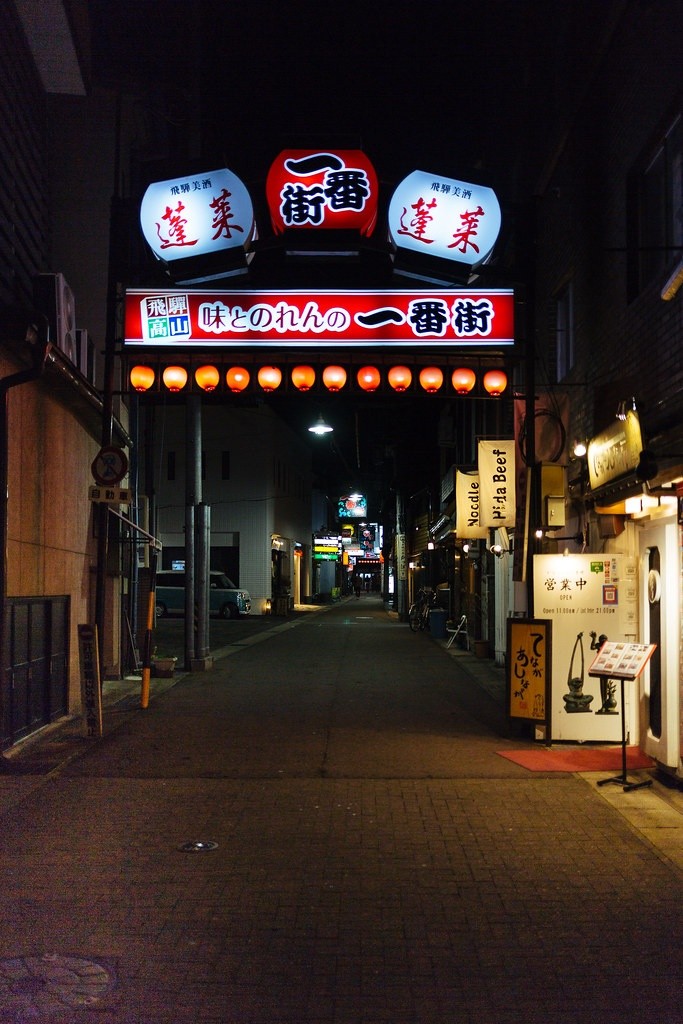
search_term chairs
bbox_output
[445,615,471,650]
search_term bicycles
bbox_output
[408,588,439,632]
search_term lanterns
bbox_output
[131,365,508,396]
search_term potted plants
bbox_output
[153,653,179,677]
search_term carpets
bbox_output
[493,744,659,774]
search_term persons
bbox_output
[354,573,362,599]
[365,574,381,593]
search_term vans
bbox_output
[155,570,253,621]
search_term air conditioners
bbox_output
[37,271,77,367]
[74,328,98,386]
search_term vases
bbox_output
[472,638,490,658]
